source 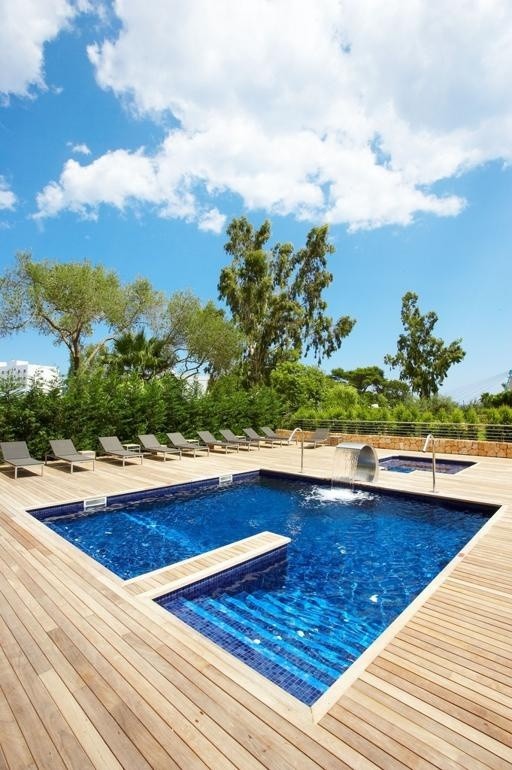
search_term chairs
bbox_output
[301,428,331,449]
[0,426,298,479]
